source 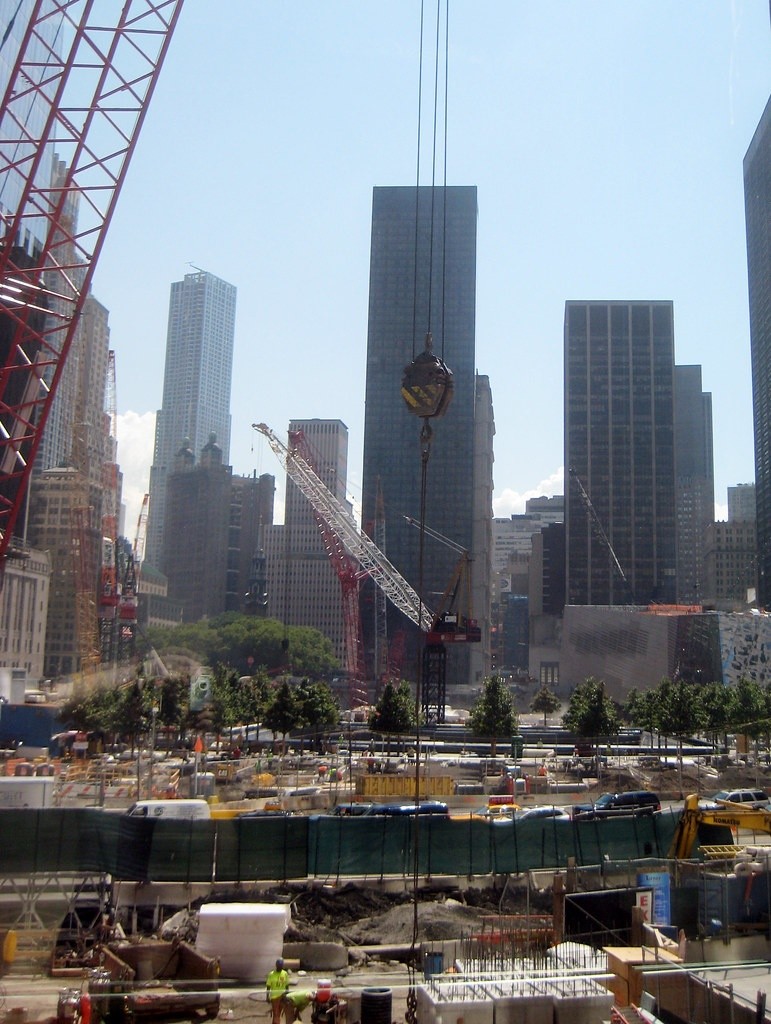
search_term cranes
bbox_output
[371,470,389,683]
[1,0,458,984]
[250,422,482,729]
[67,310,152,695]
[286,426,407,711]
[567,468,642,606]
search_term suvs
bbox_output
[331,802,384,818]
[573,790,662,821]
[699,788,771,812]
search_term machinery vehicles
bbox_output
[666,792,770,887]
[199,753,238,784]
[482,765,532,796]
[98,939,221,1024]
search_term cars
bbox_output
[474,795,529,822]
[493,804,571,826]
[233,801,298,817]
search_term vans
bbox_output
[123,799,211,820]
[362,799,450,821]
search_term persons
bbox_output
[266,957,292,1023]
[283,992,314,1024]
